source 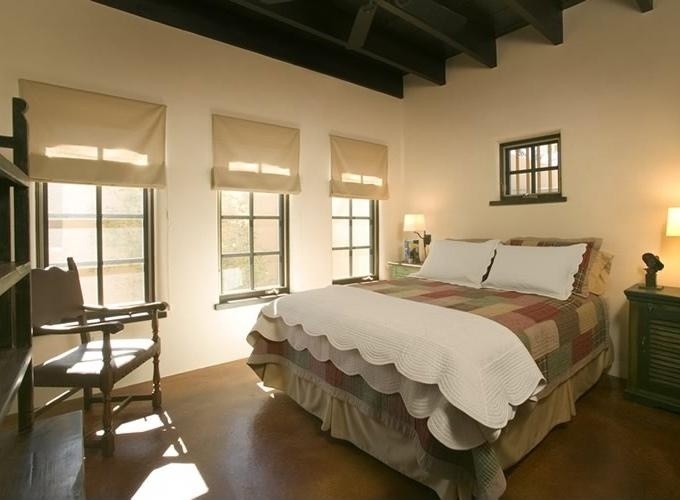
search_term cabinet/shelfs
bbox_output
[0,93,32,457]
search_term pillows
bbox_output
[416,235,612,299]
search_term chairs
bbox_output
[18,256,167,461]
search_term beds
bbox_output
[247,278,613,456]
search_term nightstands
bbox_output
[387,262,422,280]
[618,284,680,412]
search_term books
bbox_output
[403,238,419,265]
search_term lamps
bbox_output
[666,207,679,237]
[404,214,426,240]
